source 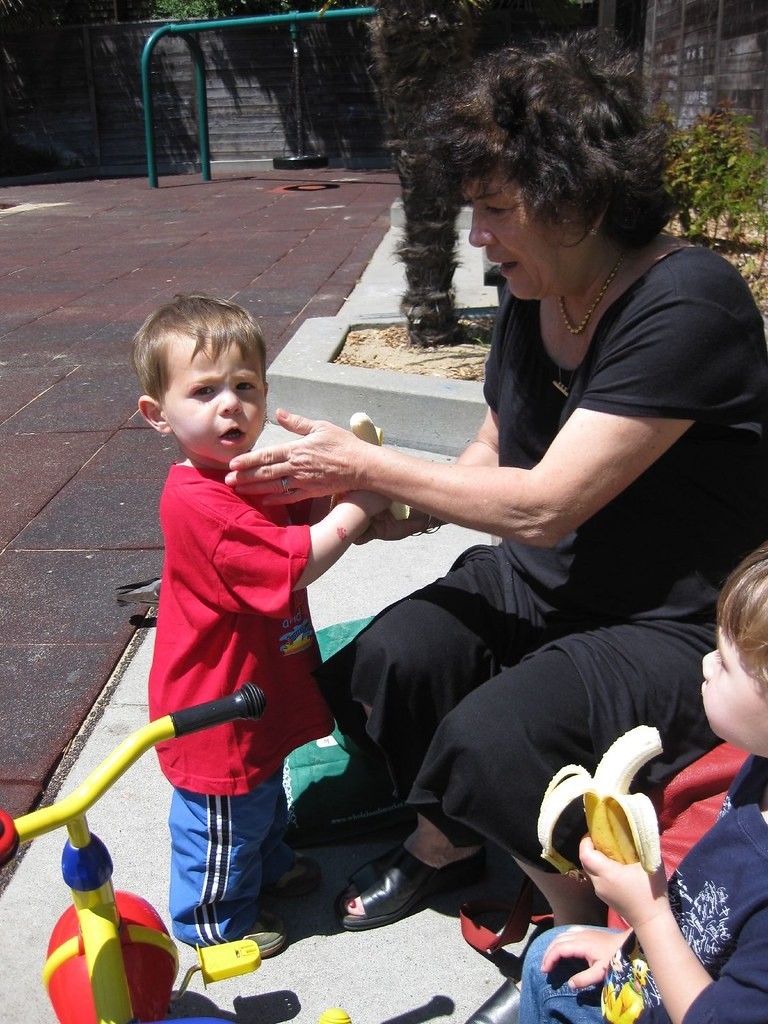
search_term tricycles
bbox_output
[0,682,268,1024]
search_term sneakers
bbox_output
[185,911,285,959]
[261,856,321,897]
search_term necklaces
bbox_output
[558,252,626,335]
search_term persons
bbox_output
[130,287,395,961]
[224,22,767,929]
[518,546,768,1024]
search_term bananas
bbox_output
[536,725,668,875]
[328,411,412,522]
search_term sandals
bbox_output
[339,841,487,932]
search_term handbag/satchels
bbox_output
[607,743,758,934]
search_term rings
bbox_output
[280,477,295,495]
